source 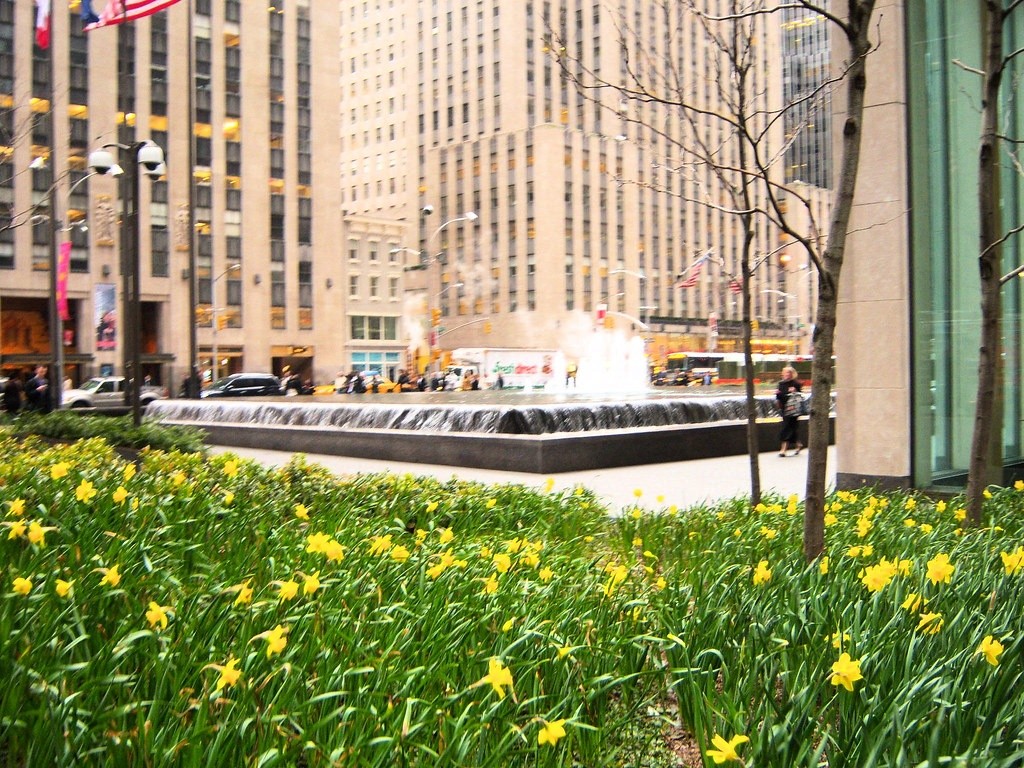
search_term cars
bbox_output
[313,371,401,394]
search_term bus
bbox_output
[716,356,836,387]
[665,353,747,386]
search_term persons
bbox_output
[394,368,479,392]
[4,372,29,416]
[334,371,385,394]
[25,362,51,416]
[279,366,315,397]
[63,376,73,391]
[650,368,713,386]
[178,371,191,399]
[776,366,804,458]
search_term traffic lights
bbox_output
[751,319,759,331]
[432,307,441,326]
[434,347,441,361]
[486,322,493,334]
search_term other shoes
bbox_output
[794,444,804,455]
[778,453,785,456]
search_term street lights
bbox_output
[212,265,241,381]
[88,139,166,425]
[389,205,478,368]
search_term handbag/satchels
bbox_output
[784,394,803,417]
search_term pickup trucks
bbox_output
[61,376,170,415]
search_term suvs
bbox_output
[201,374,283,399]
